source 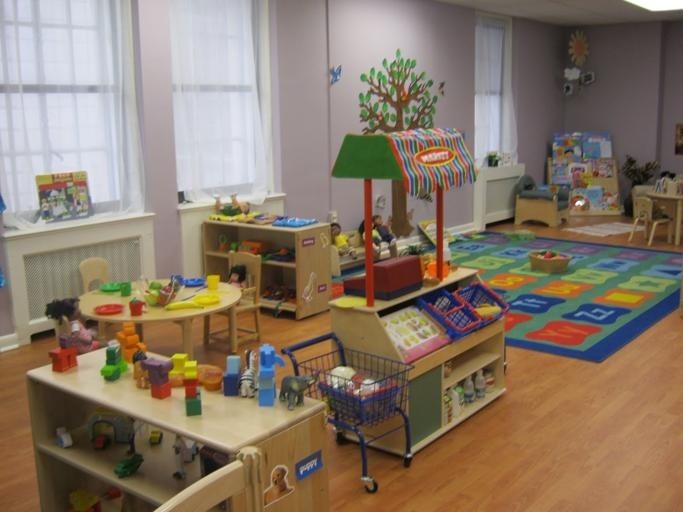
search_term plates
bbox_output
[94,303,123,315]
[97,281,120,292]
[192,294,223,305]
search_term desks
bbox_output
[644,192,683,246]
[78,278,243,354]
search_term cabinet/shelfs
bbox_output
[26,342,331,512]
[200,214,331,321]
[331,130,510,461]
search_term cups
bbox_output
[129,300,145,318]
[178,191,185,206]
[118,281,132,296]
[206,275,220,290]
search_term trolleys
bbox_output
[280,331,414,493]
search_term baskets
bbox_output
[415,282,511,342]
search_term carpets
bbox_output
[329,231,683,364]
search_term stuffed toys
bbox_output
[213,192,250,216]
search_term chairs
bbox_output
[513,174,572,228]
[203,250,262,353]
[78,257,122,342]
[627,197,673,246]
[154,445,265,512]
[52,314,72,348]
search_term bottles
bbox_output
[487,152,514,167]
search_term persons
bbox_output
[359,220,383,257]
[228,265,246,305]
[373,215,395,249]
[330,223,357,260]
[46,298,106,354]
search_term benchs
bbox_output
[330,229,398,277]
